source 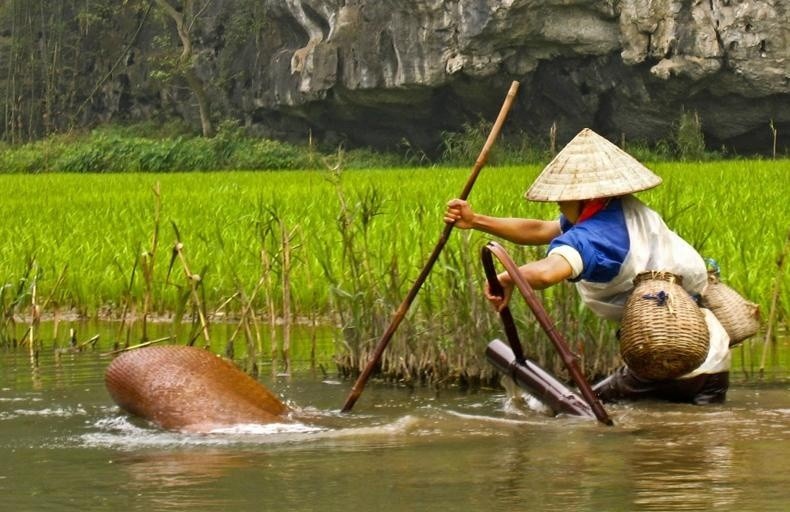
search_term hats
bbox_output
[524,127,663,203]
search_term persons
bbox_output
[444,126,732,409]
